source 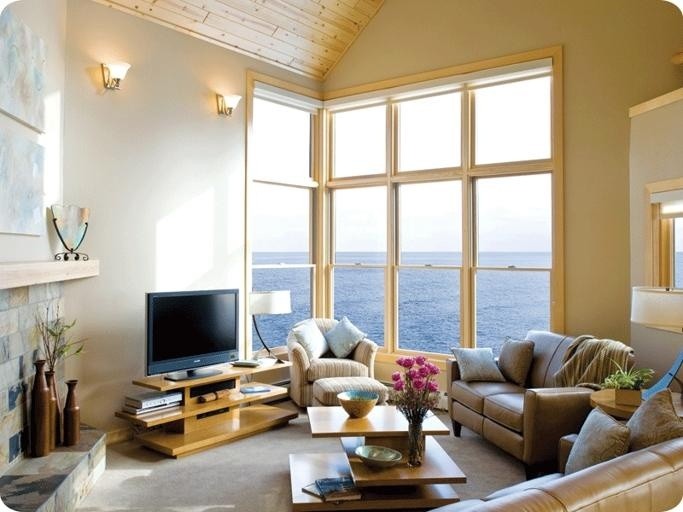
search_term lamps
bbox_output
[630,285,681,339]
[246,289,287,362]
[213,91,242,118]
[97,62,131,90]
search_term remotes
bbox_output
[233,362,260,367]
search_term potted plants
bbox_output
[599,354,655,407]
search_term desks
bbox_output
[588,388,682,424]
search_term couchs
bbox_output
[425,426,682,511]
[446,327,638,482]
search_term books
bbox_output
[240,386,271,394]
[301,476,361,504]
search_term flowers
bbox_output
[391,355,444,458]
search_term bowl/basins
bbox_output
[336,389,380,419]
[354,445,403,468]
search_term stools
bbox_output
[311,375,388,407]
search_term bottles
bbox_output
[25,359,53,458]
[63,378,80,448]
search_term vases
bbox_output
[402,410,424,465]
[24,356,81,458]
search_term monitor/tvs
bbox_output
[145,289,239,381]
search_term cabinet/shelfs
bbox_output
[113,357,298,459]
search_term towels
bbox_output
[551,332,634,389]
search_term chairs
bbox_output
[285,318,379,409]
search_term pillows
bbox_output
[292,320,329,363]
[450,345,505,384]
[498,335,536,388]
[621,389,682,452]
[564,403,631,476]
[324,316,366,358]
